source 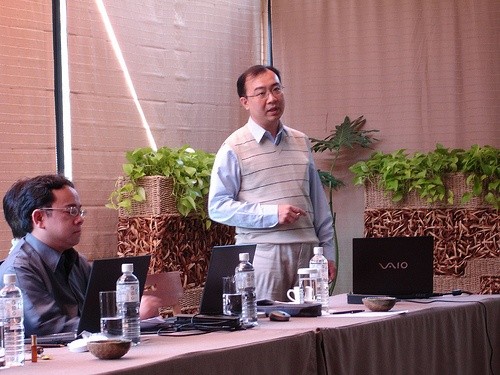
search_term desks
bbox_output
[0,293,500,375]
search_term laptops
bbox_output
[24,254,152,345]
[164,243,258,325]
[351,235,443,300]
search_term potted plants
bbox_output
[348,144,500,209]
[106,147,217,230]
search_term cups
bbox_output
[0,308,6,367]
[222,277,242,316]
[287,286,313,303]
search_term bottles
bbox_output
[100,292,123,339]
[0,274,25,366]
[298,268,317,304]
[234,253,257,328]
[116,264,140,346]
[310,247,329,316]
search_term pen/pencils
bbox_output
[330,310,365,314]
[37,344,64,348]
[297,210,308,216]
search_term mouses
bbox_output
[269,311,290,321]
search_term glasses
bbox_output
[32,207,88,219]
[242,86,286,98]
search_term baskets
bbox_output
[117,176,209,219]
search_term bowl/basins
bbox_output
[363,297,396,312]
[87,340,132,359]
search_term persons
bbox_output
[208,64,337,303]
[0,175,164,338]
